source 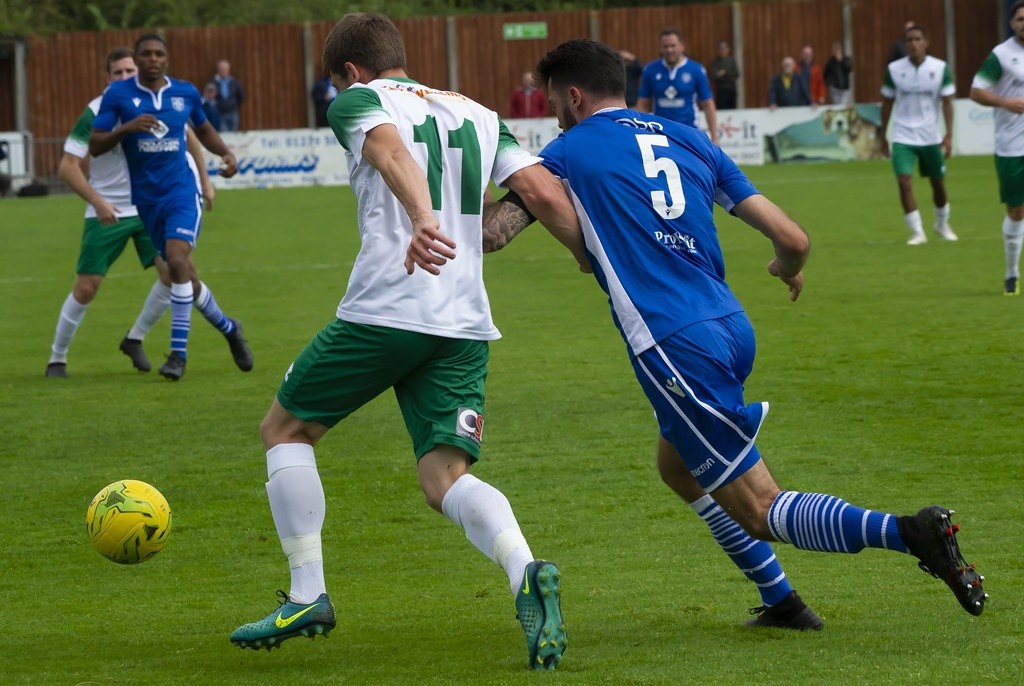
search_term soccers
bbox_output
[86,479,173,564]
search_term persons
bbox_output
[481,20,993,630]
[90,33,255,379]
[230,14,593,672]
[970,0,1024,295]
[45,48,173,381]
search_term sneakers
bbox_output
[160,354,186,379]
[934,223,958,241]
[913,506,988,615]
[230,590,337,651]
[745,590,823,632]
[515,559,569,672]
[223,319,254,372]
[907,235,928,246]
[46,362,68,378]
[119,336,151,372]
[1004,271,1020,296]
